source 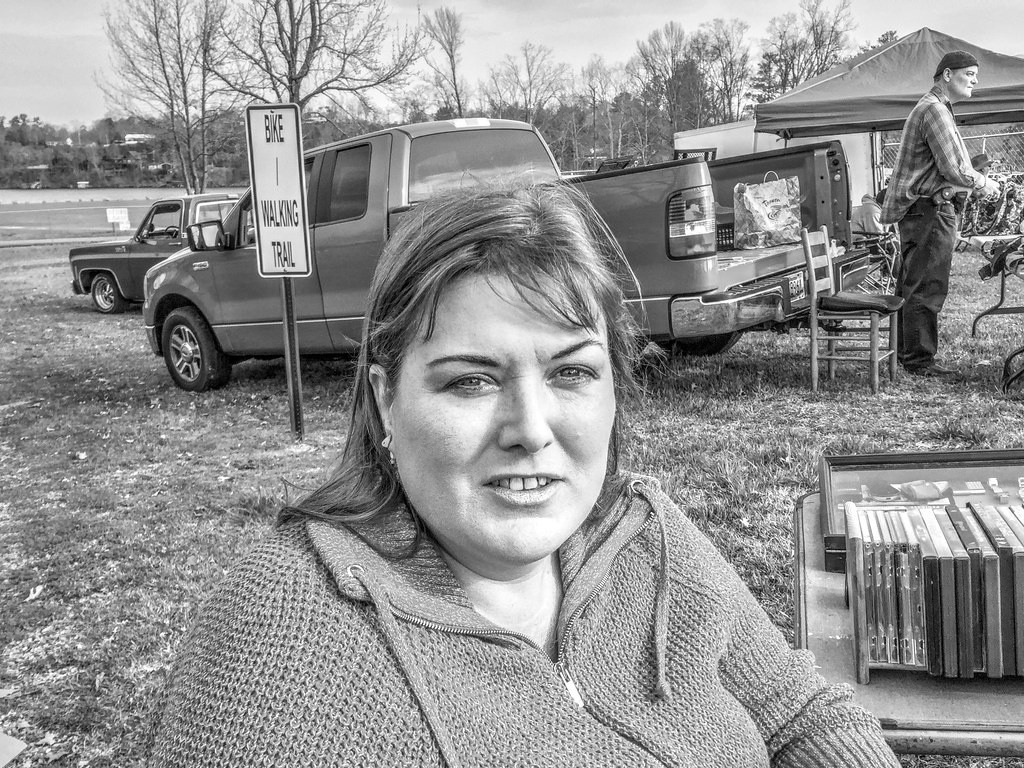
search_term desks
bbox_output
[957,230,1024,395]
[793,492,1024,757]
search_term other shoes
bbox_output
[907,363,965,384]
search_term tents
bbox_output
[754,28,1024,196]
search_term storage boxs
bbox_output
[716,223,734,251]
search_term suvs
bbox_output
[69,195,241,314]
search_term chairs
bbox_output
[800,226,904,394]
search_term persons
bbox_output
[851,51,1001,376]
[149,170,905,767]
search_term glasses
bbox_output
[987,164,991,167]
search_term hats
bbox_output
[933,51,979,78]
[970,154,994,172]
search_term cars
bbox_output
[142,117,853,393]
